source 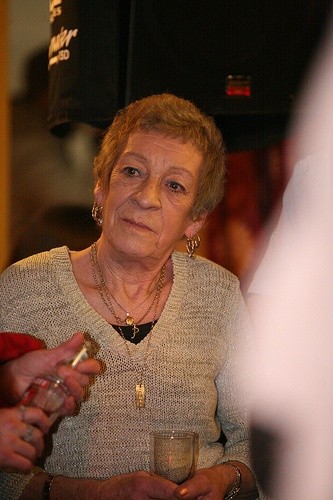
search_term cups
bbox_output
[149,431,199,486]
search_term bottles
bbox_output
[15,334,100,442]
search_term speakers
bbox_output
[87,0,333,140]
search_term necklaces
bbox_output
[89,240,166,412]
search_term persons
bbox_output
[0,95,266,500]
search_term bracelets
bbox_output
[223,460,242,500]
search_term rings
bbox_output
[19,405,26,421]
[24,425,33,439]
